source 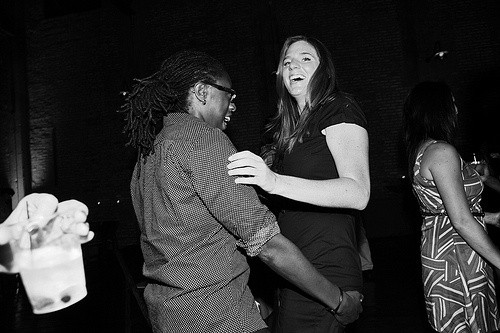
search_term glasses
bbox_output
[190,80,236,103]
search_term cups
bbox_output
[11,222,87,314]
[470,161,488,183]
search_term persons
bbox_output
[228,35,371,332]
[120,51,361,333]
[406,78,500,333]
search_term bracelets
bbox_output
[330,284,344,315]
